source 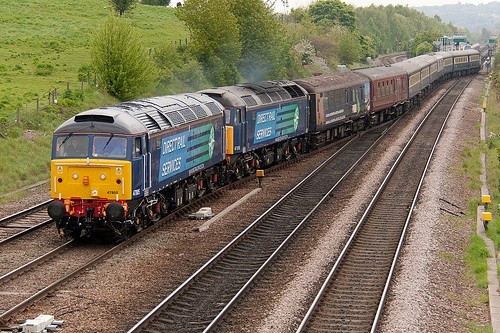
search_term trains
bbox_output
[47,50,482,242]
